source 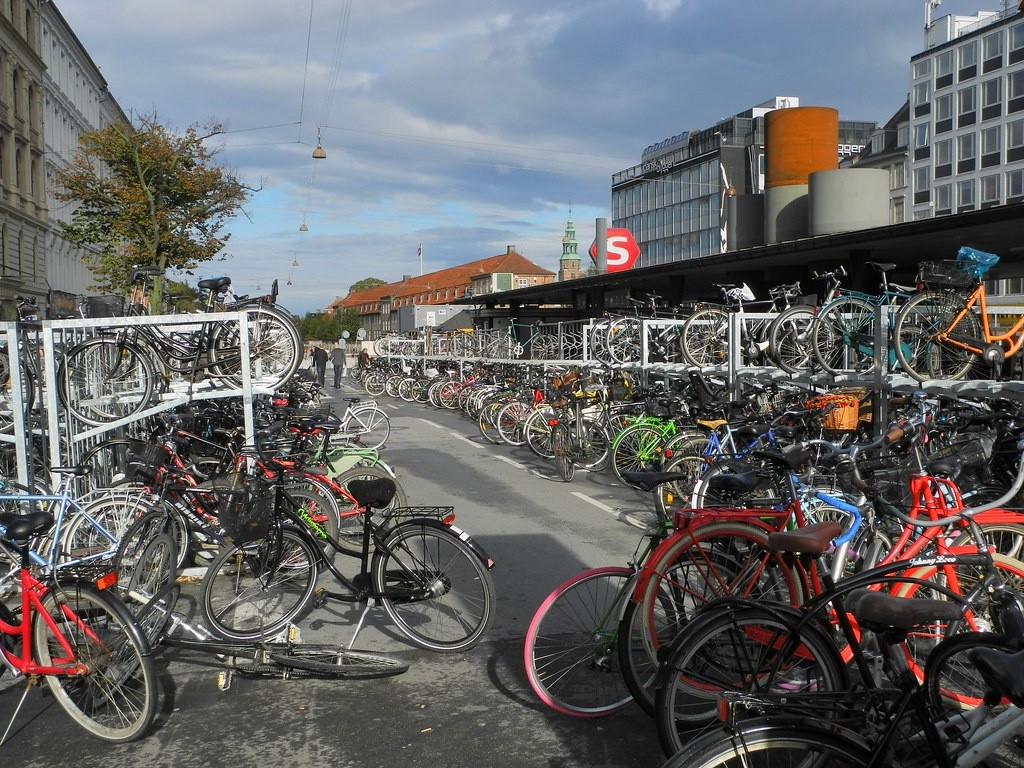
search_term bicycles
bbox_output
[57,264,312,427]
[17,259,1023,768]
[893,249,1024,385]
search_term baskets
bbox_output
[926,438,992,495]
[837,453,922,519]
[124,440,171,486]
[648,399,678,420]
[88,295,125,330]
[212,471,271,543]
[916,259,979,287]
[794,294,817,307]
[757,390,786,417]
[823,386,864,430]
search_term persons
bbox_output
[329,342,347,389]
[358,348,372,369]
[312,343,328,389]
[310,346,319,356]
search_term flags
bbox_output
[417,243,421,256]
